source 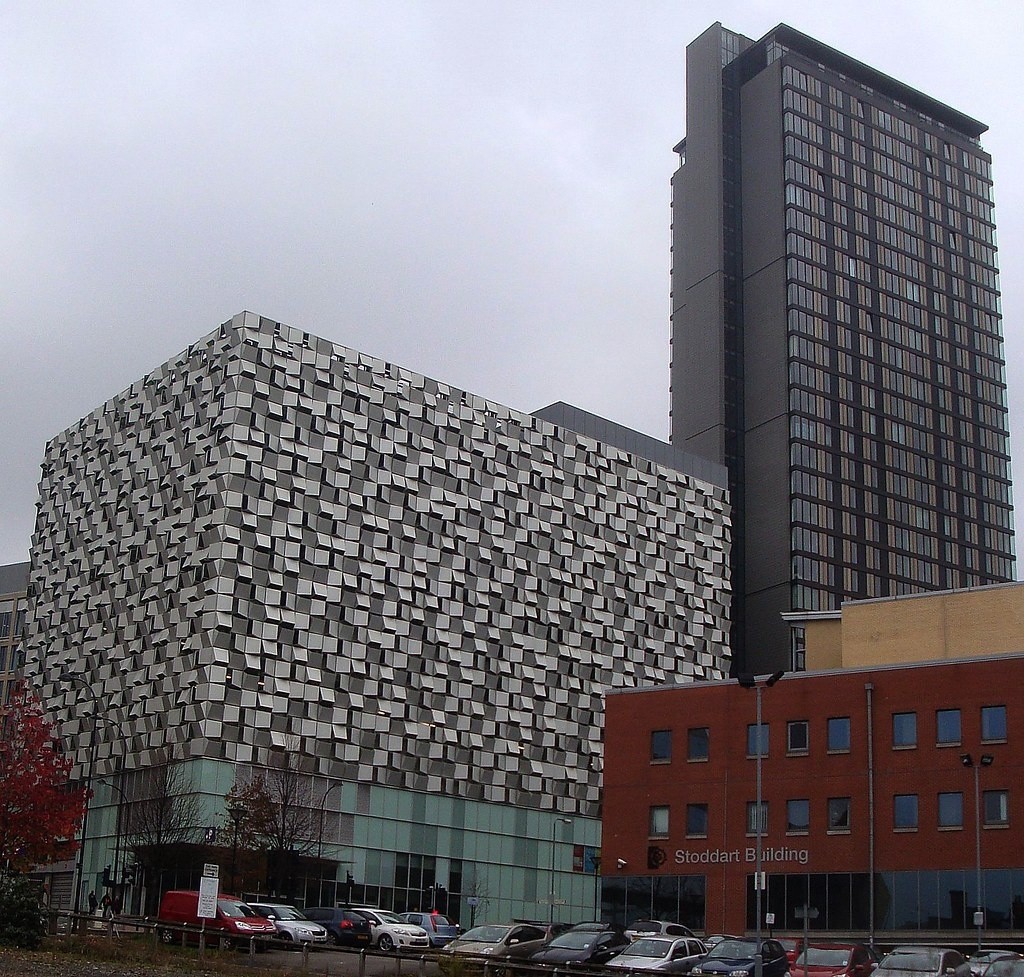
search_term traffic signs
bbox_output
[203,863,219,878]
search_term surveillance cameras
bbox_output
[617,859,627,865]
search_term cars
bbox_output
[393,912,459,949]
[439,917,624,977]
[346,907,430,953]
[783,940,885,977]
[688,932,805,977]
[295,907,373,949]
[869,945,1024,977]
[242,902,328,952]
[602,934,710,977]
[524,926,632,977]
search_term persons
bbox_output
[114,896,123,917]
[89,891,98,915]
[100,893,111,917]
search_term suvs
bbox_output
[623,918,699,945]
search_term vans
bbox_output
[157,890,277,955]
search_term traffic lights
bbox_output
[101,868,110,887]
[347,870,353,885]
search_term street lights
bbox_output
[736,670,787,977]
[315,780,344,908]
[56,673,99,934]
[551,818,572,923]
[959,752,995,952]
[90,714,128,913]
[97,778,130,914]
